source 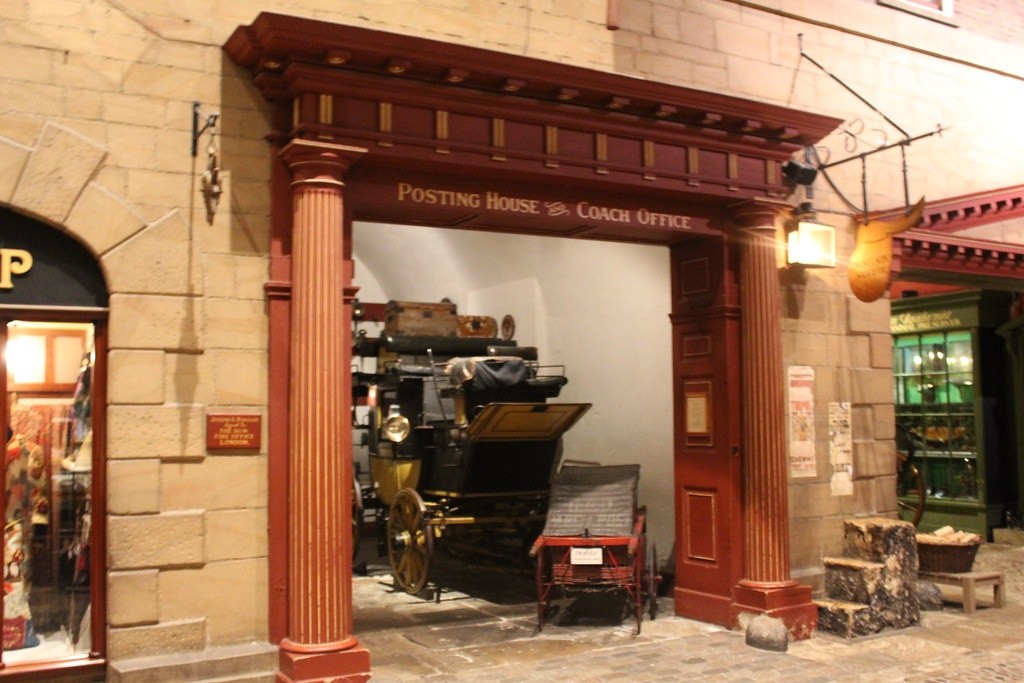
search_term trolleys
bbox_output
[530,460,667,636]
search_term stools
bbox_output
[917,567,1007,614]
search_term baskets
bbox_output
[917,534,982,574]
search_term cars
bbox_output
[351,297,596,591]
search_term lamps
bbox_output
[788,202,840,271]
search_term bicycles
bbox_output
[894,423,949,528]
